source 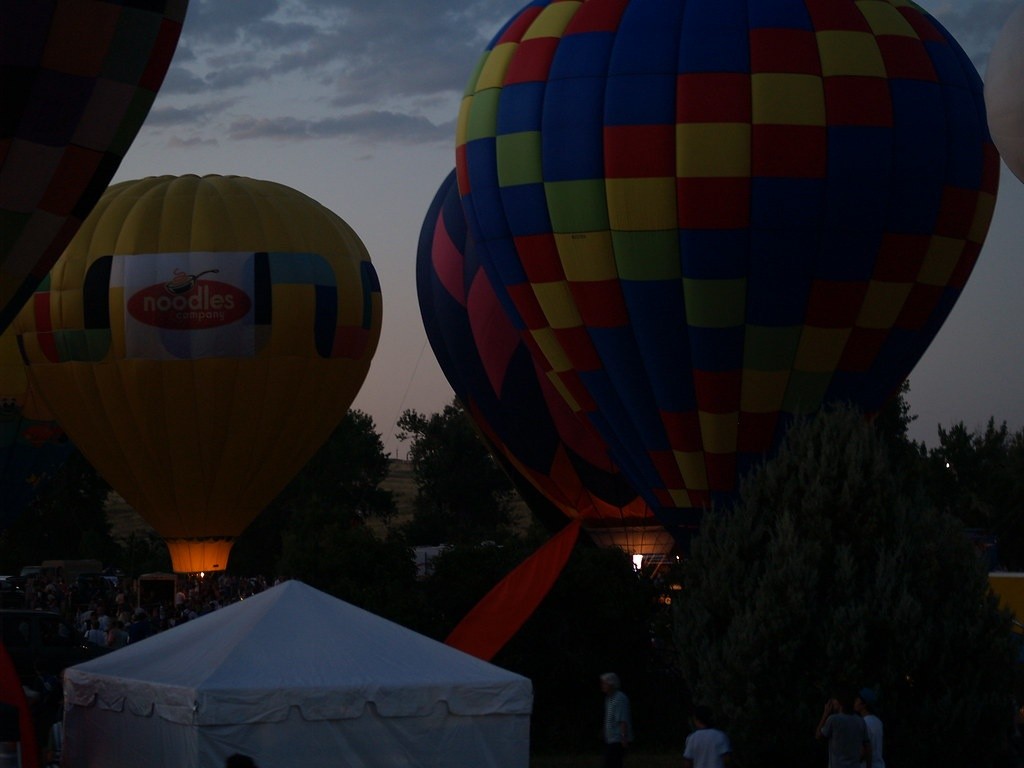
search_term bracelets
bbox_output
[620,733,627,737]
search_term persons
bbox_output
[11,562,268,659]
[227,754,258,768]
[601,673,634,768]
[815,690,886,768]
[683,705,733,768]
[46,719,63,768]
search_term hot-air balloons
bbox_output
[413,159,680,592]
[0,172,382,573]
[0,0,188,337]
[455,1,1001,573]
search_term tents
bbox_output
[61,581,533,768]
